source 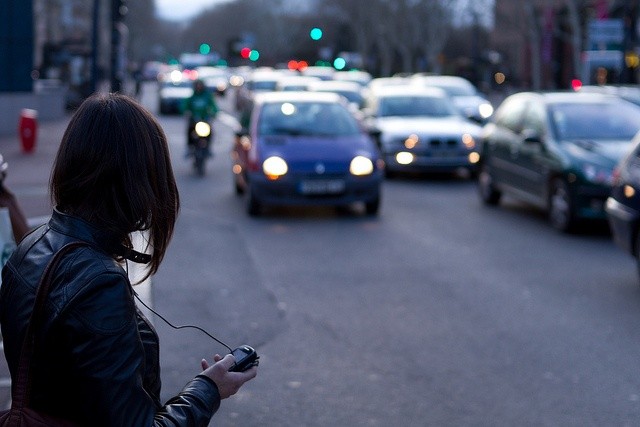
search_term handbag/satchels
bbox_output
[1,241,89,427]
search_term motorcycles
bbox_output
[188,110,213,176]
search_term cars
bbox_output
[476,91,639,233]
[231,92,383,218]
[406,74,491,123]
[196,66,373,117]
[605,129,639,272]
[160,73,195,114]
[358,80,484,182]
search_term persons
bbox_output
[1,92,259,427]
[178,79,219,159]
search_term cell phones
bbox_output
[219,344,257,372]
